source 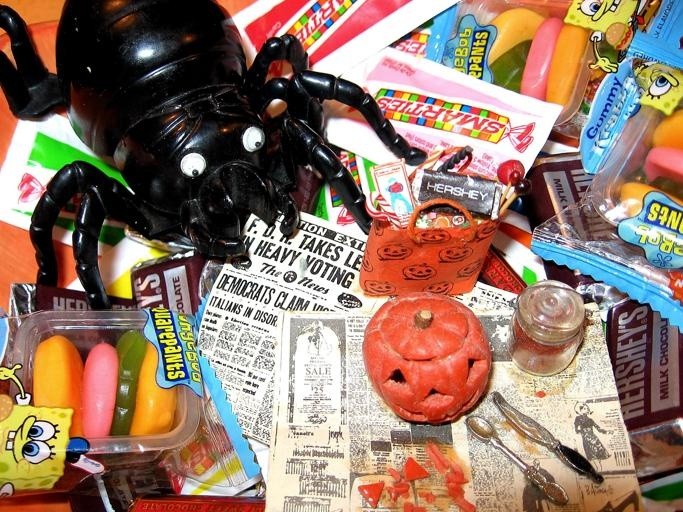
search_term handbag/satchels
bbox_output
[359,147,505,297]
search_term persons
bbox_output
[574,405,613,472]
